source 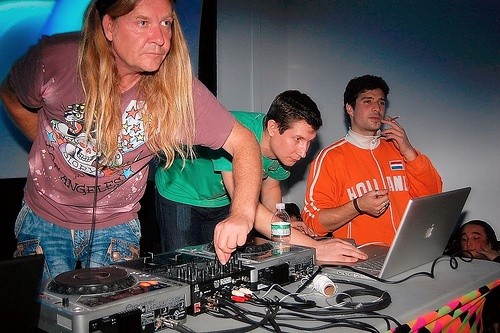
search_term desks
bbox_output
[158,254,500,333]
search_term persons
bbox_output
[284,202,303,224]
[0,0,263,287]
[155,89,368,262]
[301,74,442,247]
[453,220,500,263]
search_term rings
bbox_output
[383,202,387,208]
[374,189,378,197]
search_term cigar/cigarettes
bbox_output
[391,116,400,121]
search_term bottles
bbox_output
[270,202,292,254]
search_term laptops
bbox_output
[340,186,472,279]
[0,254,45,333]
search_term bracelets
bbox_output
[352,197,363,215]
